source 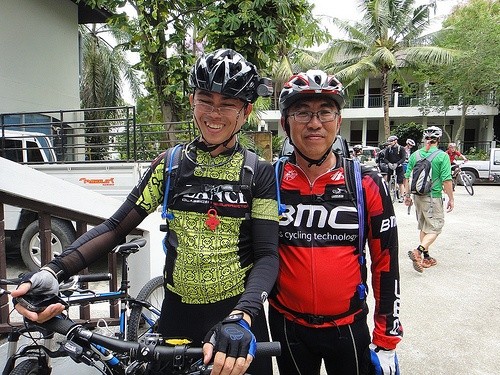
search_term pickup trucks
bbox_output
[454,147,500,185]
[1,106,274,279]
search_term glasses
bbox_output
[285,112,338,124]
[193,97,246,117]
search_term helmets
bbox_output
[278,68,346,115]
[191,49,258,103]
[353,136,457,156]
[423,126,443,138]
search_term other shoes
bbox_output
[409,249,437,272]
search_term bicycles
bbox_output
[0,233,284,375]
[377,161,472,204]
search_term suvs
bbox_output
[278,135,382,172]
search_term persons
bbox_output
[352,145,371,170]
[402,139,416,196]
[383,135,406,202]
[377,142,391,181]
[403,125,455,273]
[265,67,405,375]
[11,46,282,375]
[445,142,467,184]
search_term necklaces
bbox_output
[185,135,238,168]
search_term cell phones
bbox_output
[16,293,69,312]
